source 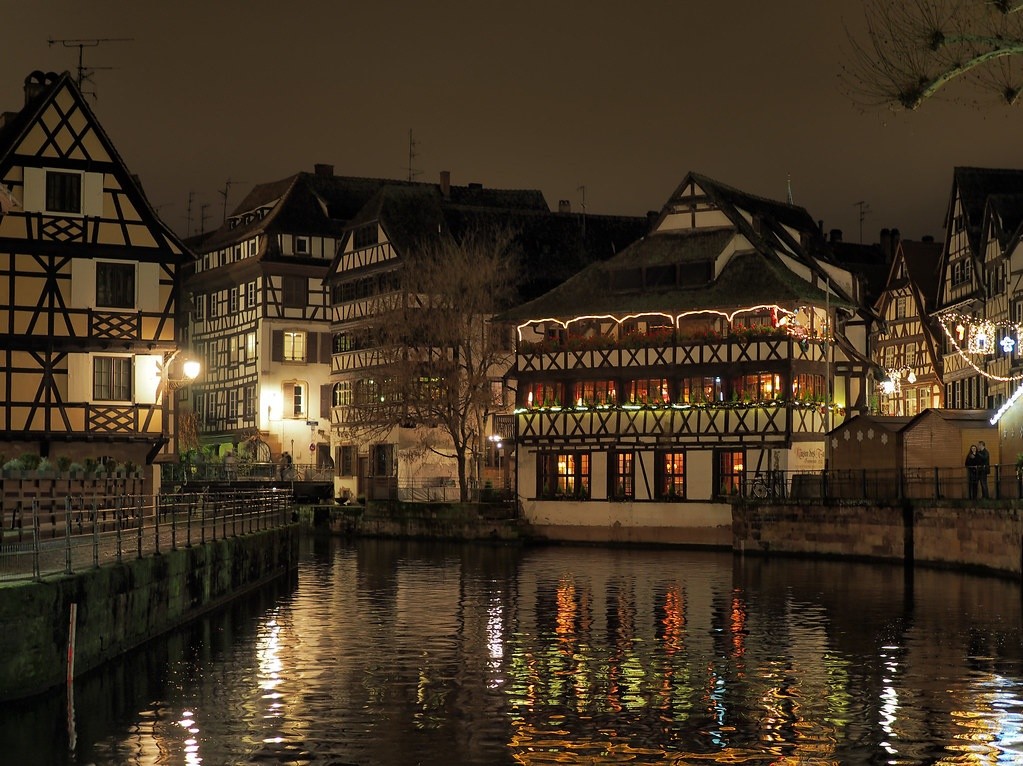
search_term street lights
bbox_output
[882,367,916,417]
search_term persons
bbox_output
[965,441,990,499]
[223,451,234,480]
[279,451,291,481]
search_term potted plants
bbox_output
[57,456,71,478]
[125,460,135,479]
[82,456,96,480]
[135,464,144,478]
[18,453,41,479]
[37,457,55,479]
[223,451,235,471]
[106,460,117,478]
[2,457,23,479]
[95,465,108,479]
[116,464,126,478]
[68,464,85,480]
